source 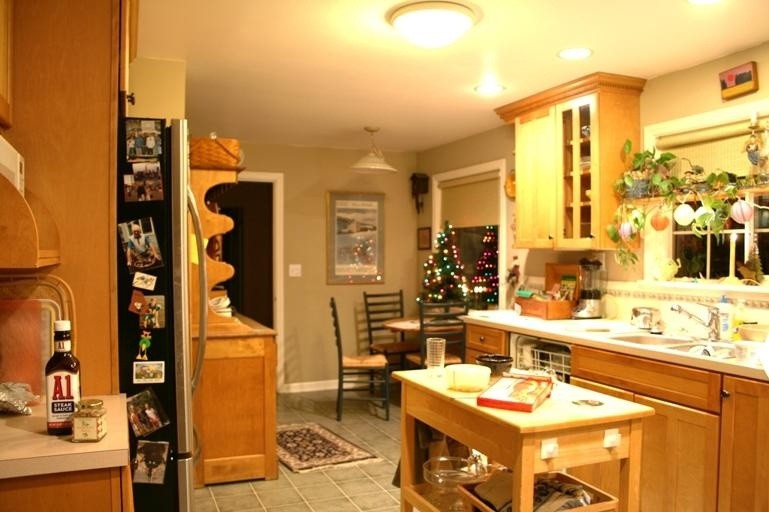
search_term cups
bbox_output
[425,338,446,378]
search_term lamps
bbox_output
[349,126,398,176]
[383,0,485,48]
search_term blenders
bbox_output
[571,258,602,318]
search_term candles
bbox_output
[729,241,736,278]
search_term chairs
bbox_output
[329,289,469,421]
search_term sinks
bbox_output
[611,335,694,345]
[652,344,741,360]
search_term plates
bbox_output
[504,169,517,201]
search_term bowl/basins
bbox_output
[422,456,487,490]
[738,324,769,342]
[631,307,660,330]
[475,353,513,377]
[444,363,492,392]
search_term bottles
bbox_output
[47,320,81,437]
[716,295,749,343]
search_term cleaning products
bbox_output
[730,300,749,341]
[719,296,730,342]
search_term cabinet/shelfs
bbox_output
[190,130,275,487]
[459,311,766,512]
[513,89,645,252]
[2,0,136,512]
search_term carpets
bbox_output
[276,421,379,473]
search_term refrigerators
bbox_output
[117,117,209,512]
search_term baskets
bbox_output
[190,136,240,171]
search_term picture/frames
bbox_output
[325,189,386,285]
[417,226,432,250]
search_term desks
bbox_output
[391,367,656,512]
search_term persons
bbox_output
[125,222,158,269]
[127,131,162,201]
[143,297,161,328]
[128,363,162,436]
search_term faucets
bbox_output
[632,306,663,334]
[671,303,720,340]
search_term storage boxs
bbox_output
[516,261,580,319]
